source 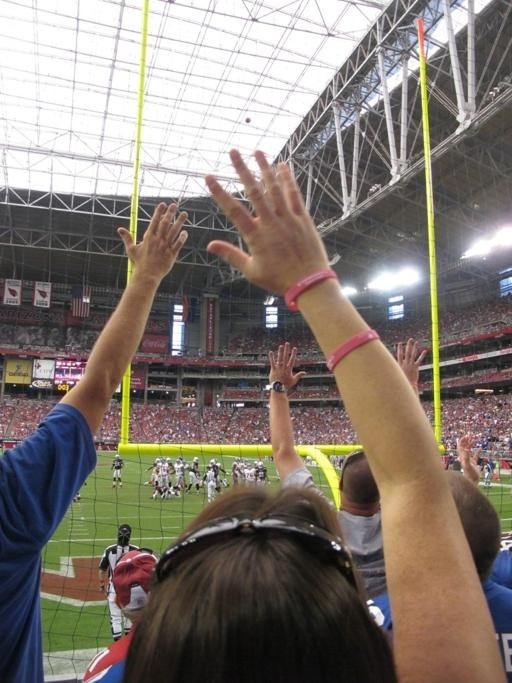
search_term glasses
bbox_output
[338,450,364,492]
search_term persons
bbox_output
[80,543,159,682]
[1,200,188,682]
[120,145,511,682]
[367,466,512,683]
[443,454,496,488]
[490,547,511,589]
[0,293,512,458]
[85,658,127,682]
[232,456,269,486]
[264,336,429,598]
[73,483,86,500]
[96,522,140,641]
[145,456,228,503]
[110,455,125,488]
[330,455,346,468]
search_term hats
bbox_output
[111,548,158,612]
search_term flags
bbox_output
[71,284,93,317]
[2,278,23,306]
[33,280,52,307]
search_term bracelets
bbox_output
[285,267,338,311]
[99,580,105,588]
